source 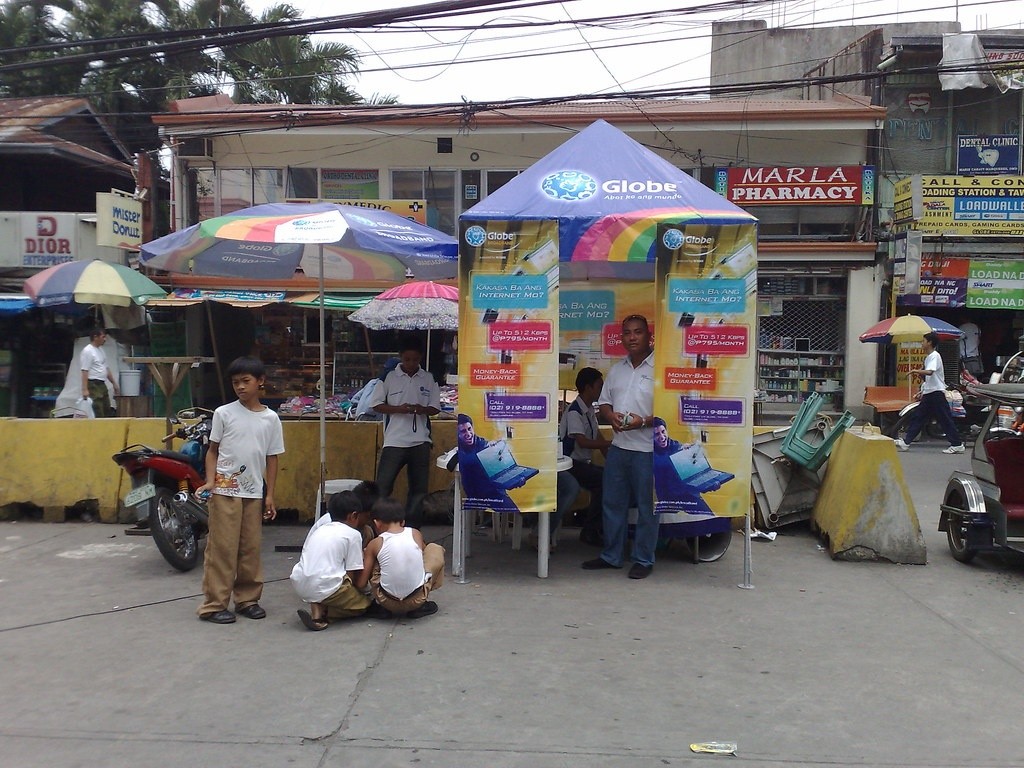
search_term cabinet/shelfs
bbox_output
[332,352,400,396]
[258,365,333,412]
[756,349,845,404]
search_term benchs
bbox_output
[986,437,1024,519]
[862,387,918,435]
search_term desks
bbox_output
[436,454,573,578]
[276,407,457,419]
[113,395,166,416]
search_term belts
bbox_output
[379,582,424,602]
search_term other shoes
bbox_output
[237,604,267,620]
[371,605,392,620]
[629,563,652,579]
[528,532,555,553]
[582,558,624,570]
[199,609,236,622]
[408,600,438,619]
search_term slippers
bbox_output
[297,609,328,631]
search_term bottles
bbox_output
[33,387,64,396]
[759,329,844,403]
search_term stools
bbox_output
[779,392,857,473]
[315,479,363,521]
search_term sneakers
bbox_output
[894,437,909,450]
[942,444,965,454]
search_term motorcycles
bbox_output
[110,406,269,572]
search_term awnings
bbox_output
[147,288,286,307]
[0,292,73,316]
[290,292,376,311]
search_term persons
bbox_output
[351,338,442,533]
[583,314,659,579]
[560,367,612,543]
[196,356,283,624]
[458,414,520,513]
[291,480,445,630]
[654,417,715,517]
[895,332,965,454]
[81,328,118,418]
[959,319,981,358]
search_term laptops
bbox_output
[476,440,539,491]
[669,441,735,493]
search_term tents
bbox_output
[459,117,758,584]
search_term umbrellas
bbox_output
[134,201,458,465]
[345,282,459,372]
[859,313,964,400]
[23,259,168,332]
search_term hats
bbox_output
[383,357,401,374]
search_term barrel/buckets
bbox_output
[120,370,141,396]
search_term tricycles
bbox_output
[936,350,1024,565]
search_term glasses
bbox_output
[623,314,647,325]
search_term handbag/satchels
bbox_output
[74,397,96,418]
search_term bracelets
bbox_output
[641,418,646,429]
